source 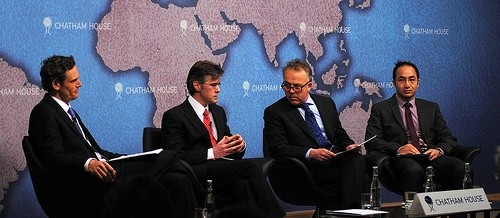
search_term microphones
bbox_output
[408,112,411,116]
[210,121,212,125]
[73,117,76,121]
[311,112,314,117]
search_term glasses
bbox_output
[199,82,221,88]
[281,80,311,92]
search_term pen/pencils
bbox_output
[330,145,334,151]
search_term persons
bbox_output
[263,60,375,210]
[365,60,467,197]
[28,56,195,218]
[161,61,286,218]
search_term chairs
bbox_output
[142,127,274,189]
[264,127,388,216]
[365,144,482,202]
[21,136,109,218]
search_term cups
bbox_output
[194,207,207,218]
[472,185,480,188]
[361,193,372,209]
[405,192,416,206]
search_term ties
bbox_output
[68,108,108,160]
[300,103,339,153]
[402,103,423,154]
[203,109,217,148]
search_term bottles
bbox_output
[463,163,473,190]
[425,167,434,192]
[204,180,215,218]
[370,166,382,209]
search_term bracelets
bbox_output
[437,149,442,156]
